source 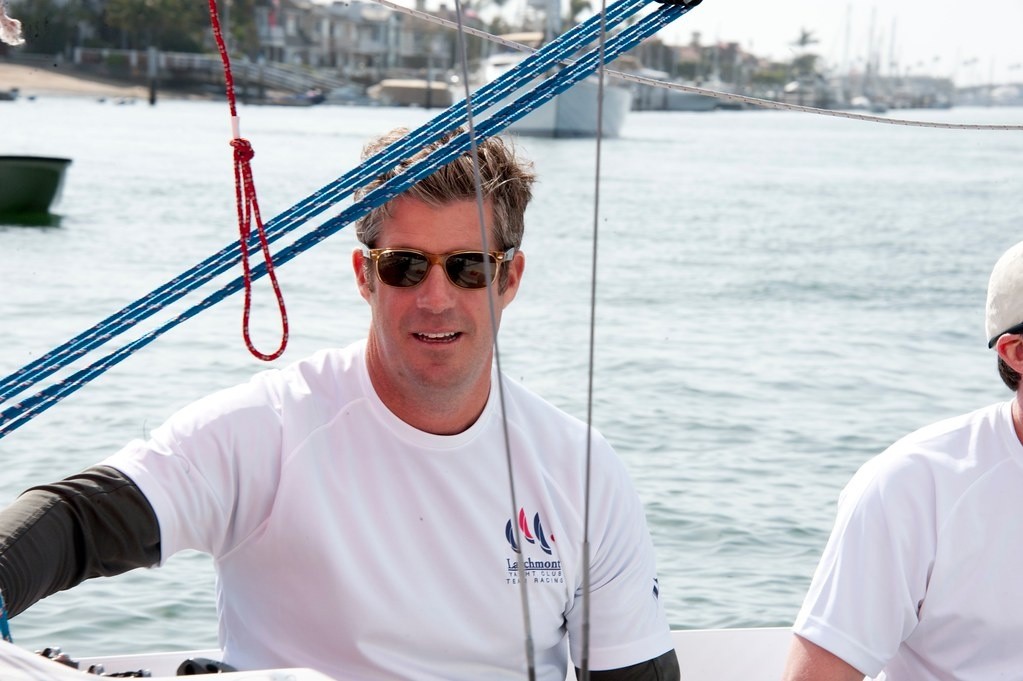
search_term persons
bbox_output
[0,119,680,681]
[780,241,1023,681]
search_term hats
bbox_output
[986,241,1023,350]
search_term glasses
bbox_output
[367,246,514,290]
